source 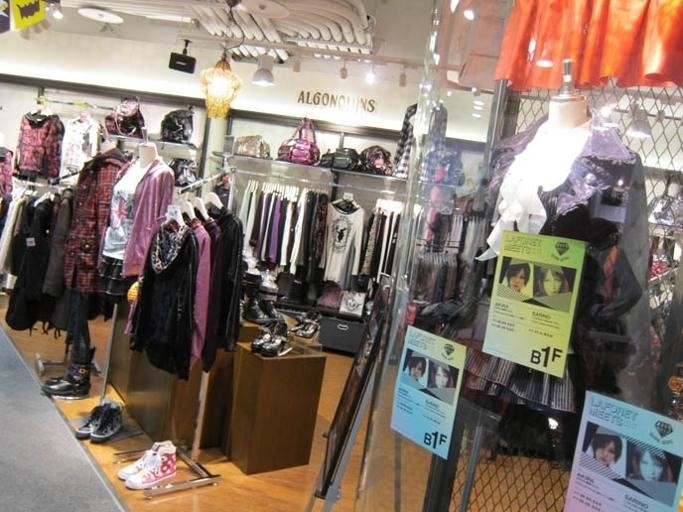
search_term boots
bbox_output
[242,295,288,356]
[41,346,97,400]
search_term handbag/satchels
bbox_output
[316,280,367,318]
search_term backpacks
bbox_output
[231,135,272,160]
[104,95,145,139]
[277,118,392,176]
[160,105,195,143]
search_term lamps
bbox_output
[624,92,666,137]
[252,54,274,86]
[293,50,410,87]
[200,0,245,120]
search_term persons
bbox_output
[505,263,530,293]
[533,264,569,297]
[590,433,622,469]
[408,356,426,382]
[627,445,674,483]
[40,140,128,400]
[93,127,175,296]
[437,58,652,470]
[426,361,454,389]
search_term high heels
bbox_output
[291,309,322,337]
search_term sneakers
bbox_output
[75,399,124,442]
[118,440,177,489]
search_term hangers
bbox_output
[325,182,366,214]
[147,175,242,246]
[21,93,104,136]
[244,169,331,204]
[369,190,426,223]
[2,178,72,212]
